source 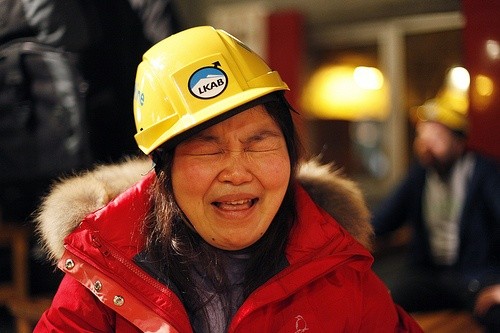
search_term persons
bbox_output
[371,83,500,333]
[32,25,425,333]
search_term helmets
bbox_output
[409,90,470,130]
[133,24,291,155]
